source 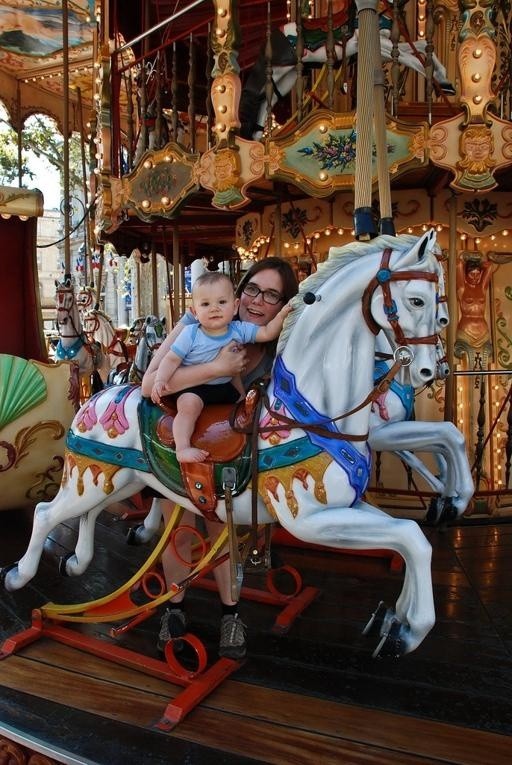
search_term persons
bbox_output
[150,271,291,463]
[142,256,299,660]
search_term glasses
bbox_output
[241,282,283,307]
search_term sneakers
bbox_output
[157,609,186,654]
[218,614,248,661]
